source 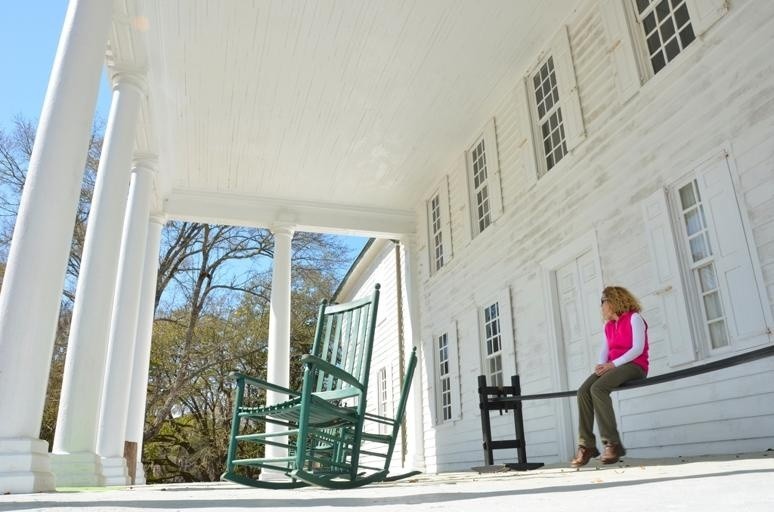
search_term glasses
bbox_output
[601,297,608,305]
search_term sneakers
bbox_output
[570,446,600,468]
[601,442,626,465]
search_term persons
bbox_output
[570,285,649,468]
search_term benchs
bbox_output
[472,345,773,473]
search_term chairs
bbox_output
[220,283,421,491]
